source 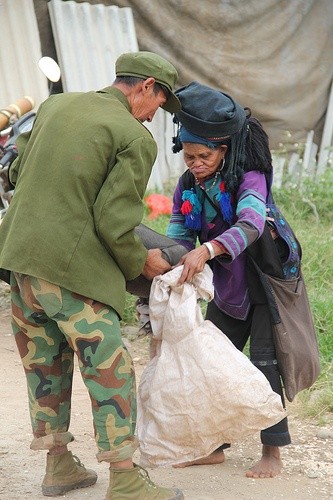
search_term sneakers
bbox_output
[42,451,97,496]
[106,463,184,500]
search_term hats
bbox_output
[115,52,182,113]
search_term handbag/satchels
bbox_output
[256,266,319,402]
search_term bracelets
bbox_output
[204,242,215,260]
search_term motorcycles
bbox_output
[0,55,61,220]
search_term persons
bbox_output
[167,84,302,478]
[0,51,184,500]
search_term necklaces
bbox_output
[194,173,218,192]
